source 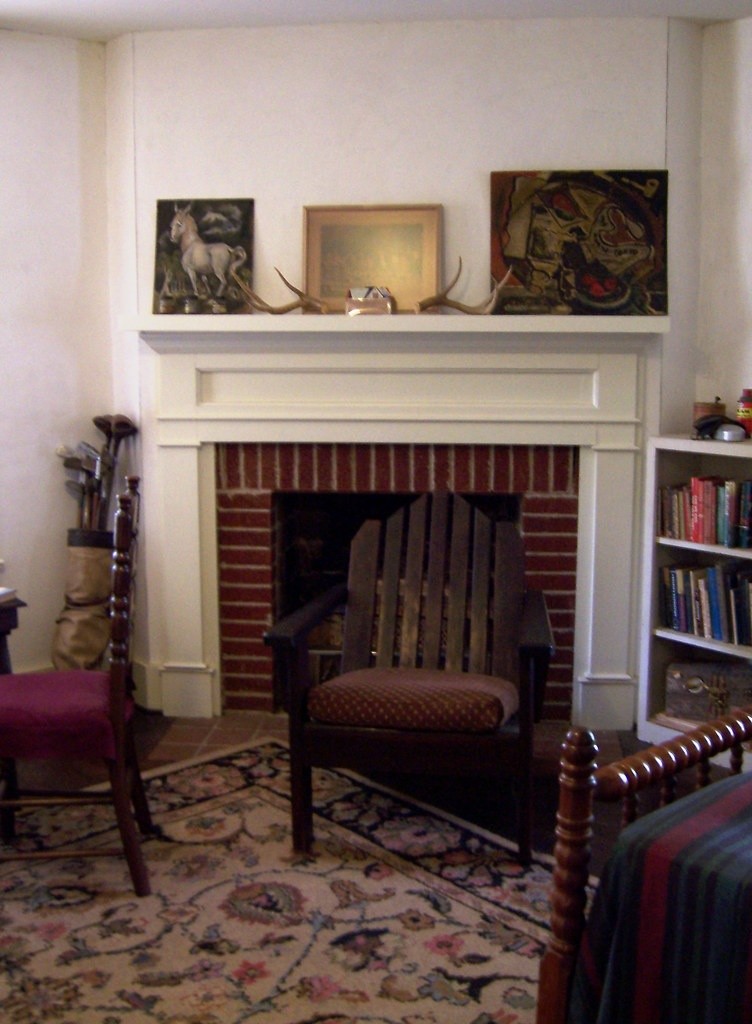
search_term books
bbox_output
[656,562,752,645]
[656,468,752,551]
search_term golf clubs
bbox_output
[56,441,100,533]
[66,480,85,530]
[92,414,139,534]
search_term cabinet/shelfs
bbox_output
[635,434,751,777]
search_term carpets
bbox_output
[0,734,604,1024]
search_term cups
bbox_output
[691,402,725,440]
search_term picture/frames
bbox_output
[301,202,445,315]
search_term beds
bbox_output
[536,706,752,1024]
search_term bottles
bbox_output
[736,389,752,438]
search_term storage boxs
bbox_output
[664,660,750,720]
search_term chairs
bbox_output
[264,496,557,870]
[1,474,155,898]
